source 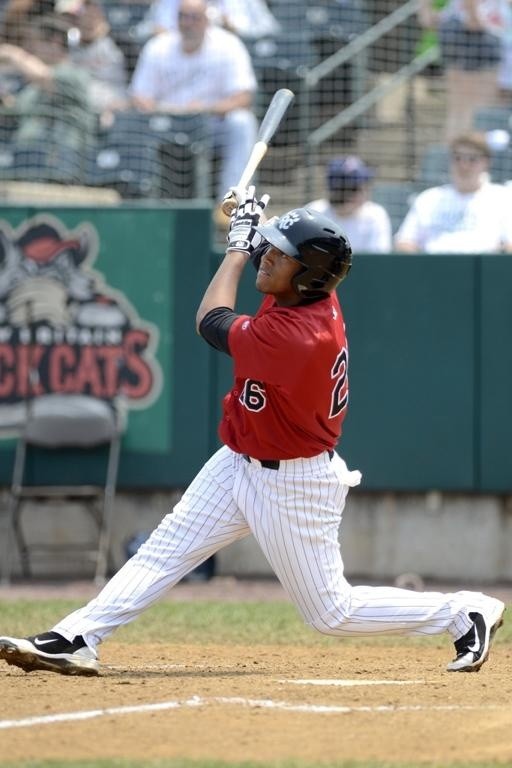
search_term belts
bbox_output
[242,450,334,471]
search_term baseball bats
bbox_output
[221,88,294,217]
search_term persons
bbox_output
[0,176,508,682]
[391,131,512,255]
[299,153,393,256]
[435,0,512,85]
[0,0,281,202]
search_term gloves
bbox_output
[226,186,274,254]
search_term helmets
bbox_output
[250,207,353,296]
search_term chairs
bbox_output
[0,0,512,233]
[1,413,120,587]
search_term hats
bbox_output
[326,153,374,207]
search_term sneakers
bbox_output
[446,590,506,672]
[0,630,100,676]
